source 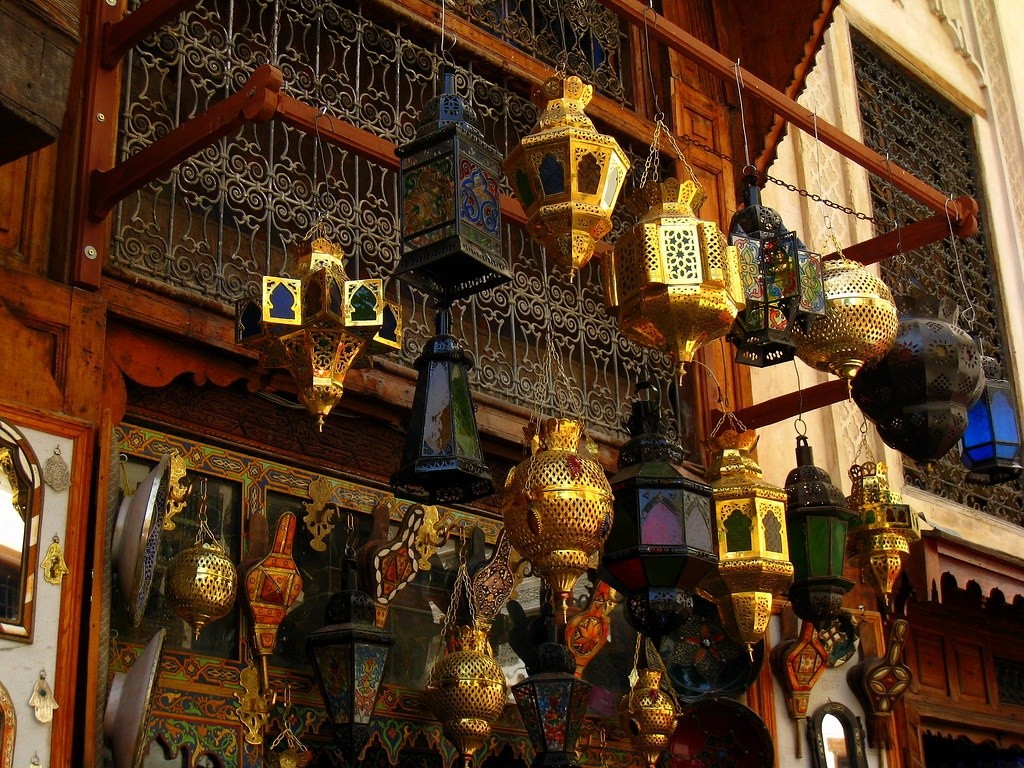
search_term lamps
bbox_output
[606,390,721,638]
[233,215,403,435]
[725,172,825,369]
[423,565,509,768]
[846,436,922,607]
[799,235,897,401]
[957,332,1024,486]
[499,74,632,283]
[708,404,795,664]
[503,385,616,625]
[782,430,860,629]
[851,291,986,478]
[389,58,514,504]
[174,474,238,642]
[617,625,682,768]
[603,114,745,386]
[303,563,396,768]
[511,608,593,768]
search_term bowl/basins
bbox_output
[103,629,167,768]
[110,452,172,629]
